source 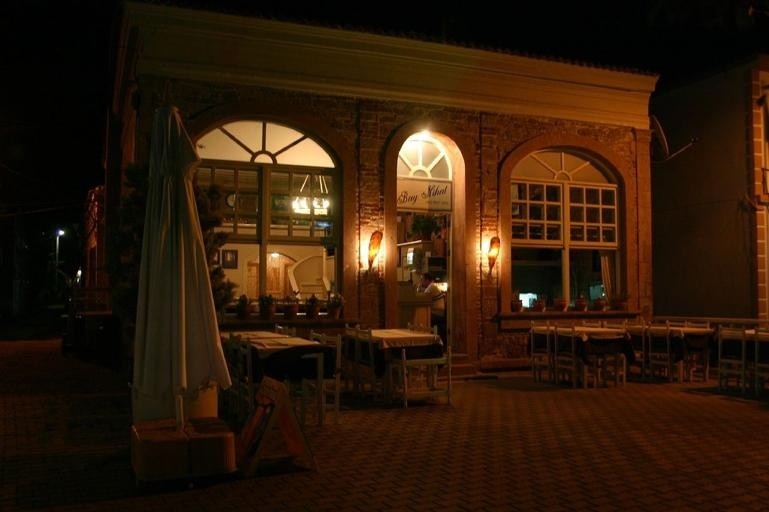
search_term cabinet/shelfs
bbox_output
[397,241,445,281]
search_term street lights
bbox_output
[55,229,65,288]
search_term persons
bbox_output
[416,273,446,327]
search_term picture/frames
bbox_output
[221,249,238,268]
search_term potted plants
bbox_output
[407,214,441,240]
[510,286,609,312]
[235,290,347,321]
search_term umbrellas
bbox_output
[131,106,232,430]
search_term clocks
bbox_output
[225,192,243,209]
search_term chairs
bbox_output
[218,320,454,433]
[529,318,769,401]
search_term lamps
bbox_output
[287,171,333,217]
[487,236,501,277]
[366,231,383,281]
